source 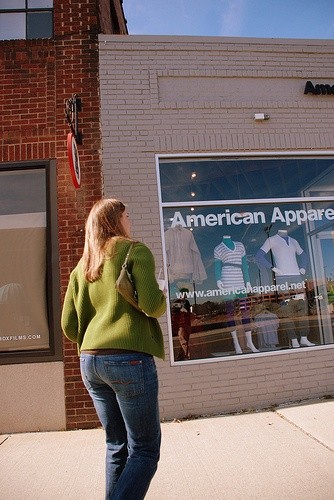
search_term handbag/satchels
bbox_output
[114,241,143,312]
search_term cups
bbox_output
[157,280,165,290]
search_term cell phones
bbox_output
[157,280,165,290]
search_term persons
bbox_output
[258,230,316,347]
[62,199,167,500]
[214,235,259,354]
